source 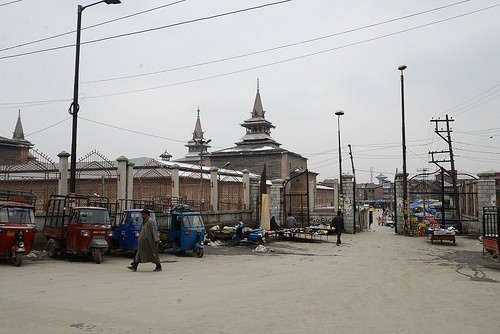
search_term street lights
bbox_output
[335,112,344,194]
[69,0,121,193]
[398,66,409,235]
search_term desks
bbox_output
[270,226,336,242]
[431,235,456,244]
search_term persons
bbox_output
[376,210,387,226]
[270,216,281,237]
[333,210,344,246]
[286,213,299,237]
[369,211,373,228]
[127,209,162,271]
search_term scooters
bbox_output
[231,216,267,246]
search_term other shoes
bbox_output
[127,266,137,272]
[336,242,339,246]
[153,268,162,271]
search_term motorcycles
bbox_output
[149,195,206,258]
[105,197,158,258]
[42,194,114,264]
[0,189,37,267]
